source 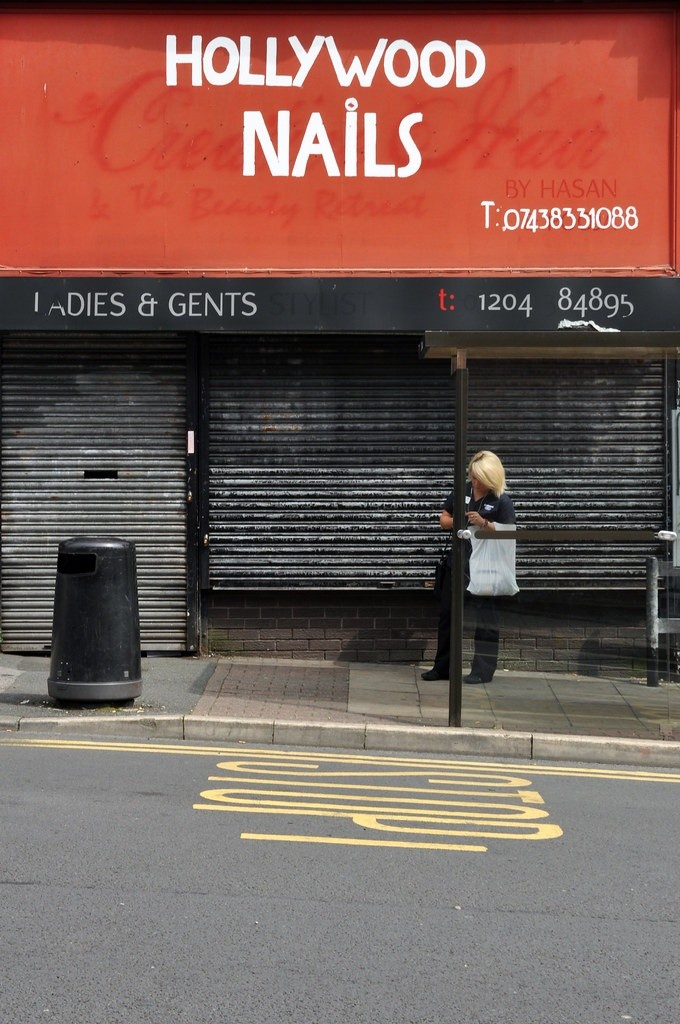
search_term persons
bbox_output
[421,449,516,685]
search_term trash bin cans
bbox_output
[47,537,142,708]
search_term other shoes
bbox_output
[421,668,450,681]
[463,674,494,684]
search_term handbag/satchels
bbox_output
[434,558,447,600]
[465,521,520,597]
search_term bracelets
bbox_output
[480,521,484,529]
[483,519,488,529]
